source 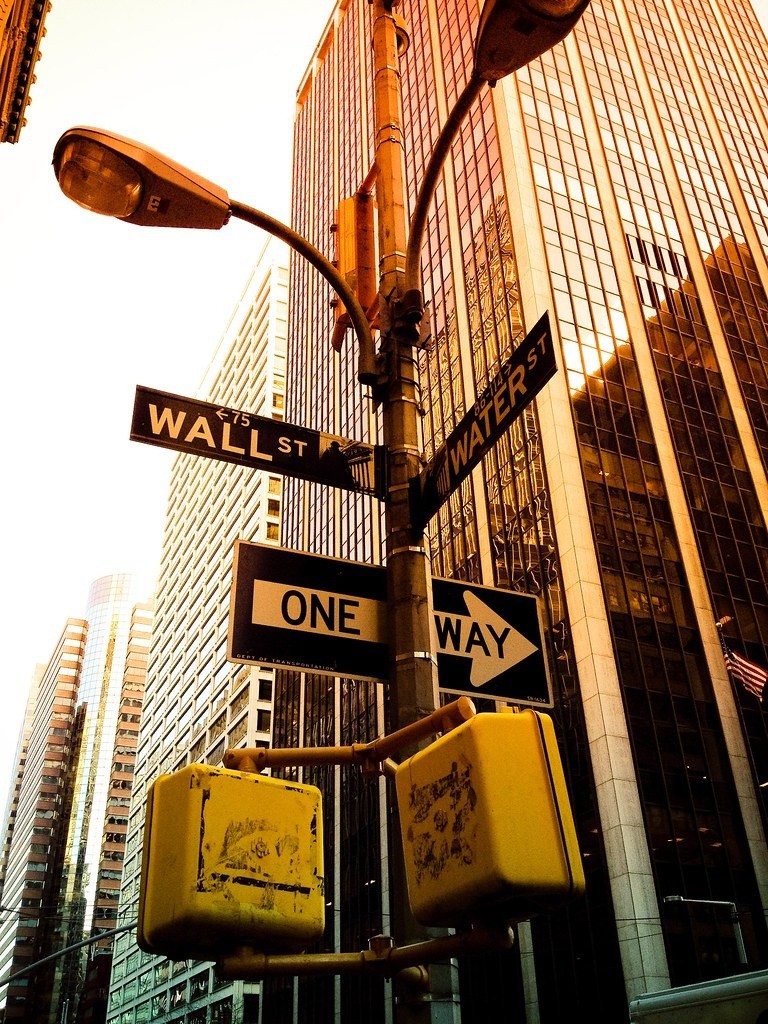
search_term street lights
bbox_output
[50,1,594,1024]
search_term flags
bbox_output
[723,645,768,702]
